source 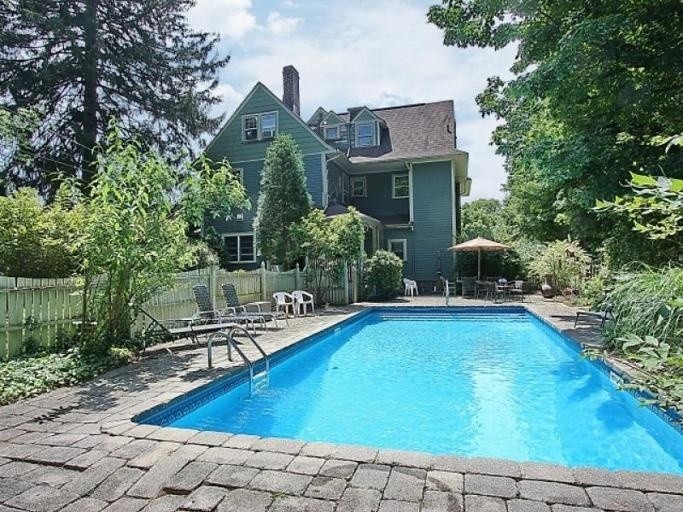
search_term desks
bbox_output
[245,302,271,321]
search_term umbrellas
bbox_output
[447,235,512,282]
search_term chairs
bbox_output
[436,275,526,304]
[270,291,296,319]
[185,284,268,340]
[290,290,316,315]
[402,276,419,297]
[221,283,290,332]
